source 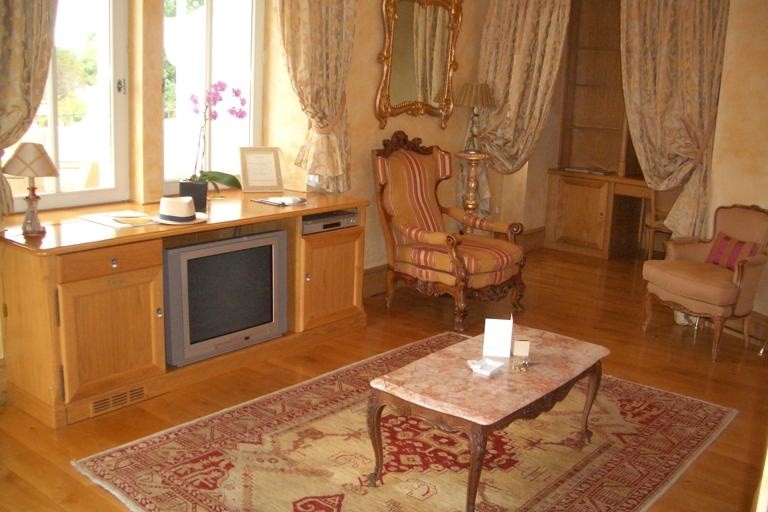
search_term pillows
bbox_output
[705,231,760,271]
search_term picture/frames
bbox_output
[240,146,284,193]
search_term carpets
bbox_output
[69,331,740,512]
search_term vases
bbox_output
[179,179,208,213]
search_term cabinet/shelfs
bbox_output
[1,190,371,430]
[543,1,685,262]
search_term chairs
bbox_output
[371,130,528,332]
[642,203,768,364]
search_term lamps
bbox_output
[1,142,60,238]
[454,82,498,235]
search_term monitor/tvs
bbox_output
[163,228,289,369]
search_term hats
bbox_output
[151,195,208,225]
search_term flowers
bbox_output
[189,81,247,193]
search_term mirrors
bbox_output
[374,1,463,130]
